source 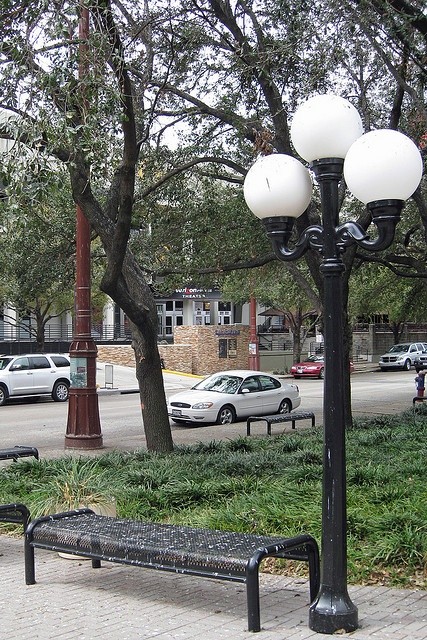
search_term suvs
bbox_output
[414,350,427,372]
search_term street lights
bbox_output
[243,96,423,636]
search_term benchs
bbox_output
[1,502,32,586]
[412,395,425,407]
[248,412,316,437]
[25,507,321,633]
[1,446,42,464]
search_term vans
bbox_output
[379,341,427,370]
[0,350,72,403]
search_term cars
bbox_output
[292,355,356,377]
[163,369,303,426]
